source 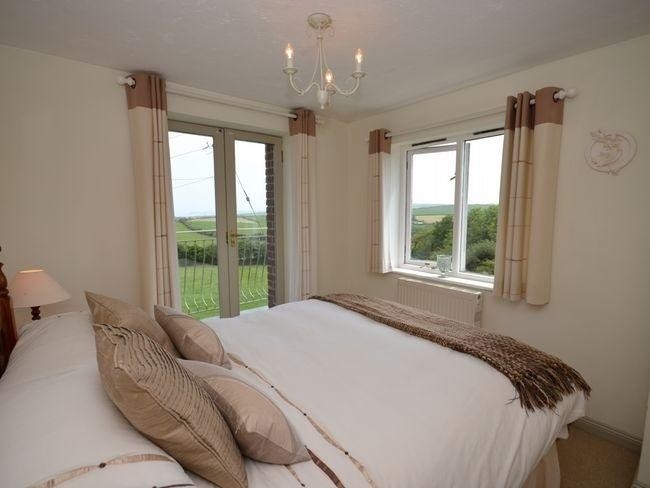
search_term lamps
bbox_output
[281,12,367,112]
[6,268,73,323]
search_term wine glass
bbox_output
[436,254,452,279]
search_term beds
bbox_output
[1,244,589,487]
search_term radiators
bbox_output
[396,275,484,329]
